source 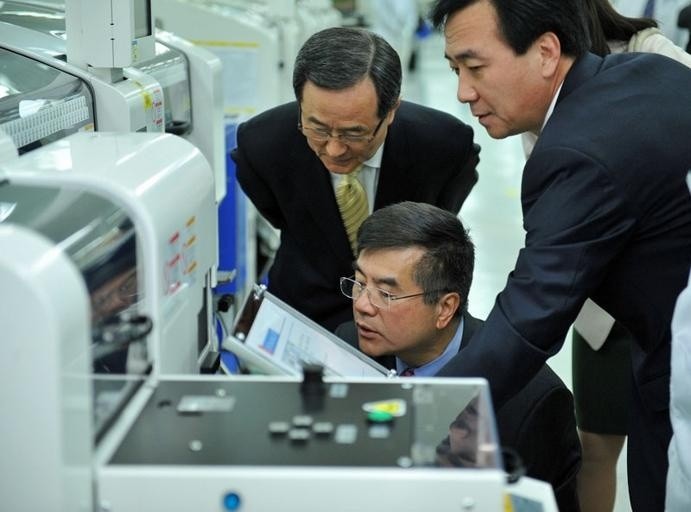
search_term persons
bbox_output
[81,230,149,399]
[228,25,480,326]
[570,1,691,512]
[424,0,691,512]
[420,385,499,470]
[333,200,581,512]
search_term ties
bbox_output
[335,171,370,259]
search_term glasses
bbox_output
[339,274,450,310]
[296,104,388,145]
